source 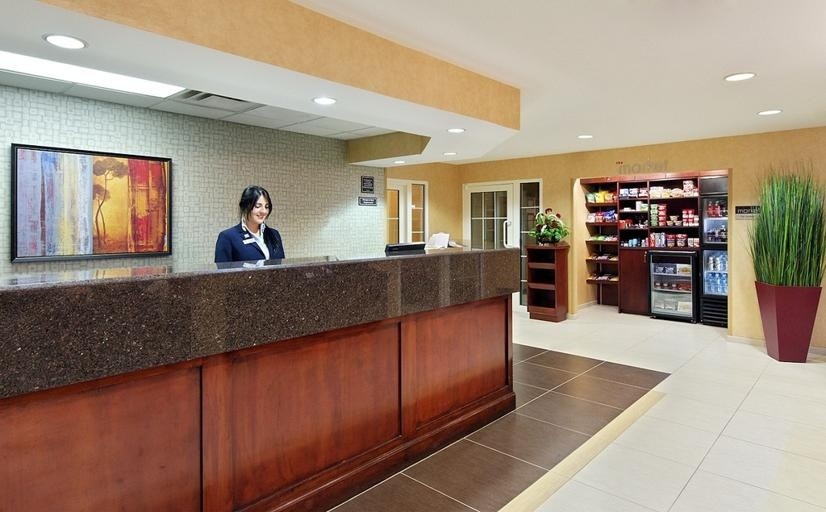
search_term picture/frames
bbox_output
[11,142,171,266]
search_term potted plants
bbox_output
[745,156,826,366]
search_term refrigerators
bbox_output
[648,250,698,324]
[700,177,729,328]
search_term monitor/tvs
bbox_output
[385,242,426,251]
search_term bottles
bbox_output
[705,200,728,294]
[655,281,679,291]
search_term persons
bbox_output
[212,185,286,263]
[215,258,282,268]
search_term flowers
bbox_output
[529,208,567,239]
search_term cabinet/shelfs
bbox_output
[580,175,650,316]
[525,244,571,323]
[648,172,699,252]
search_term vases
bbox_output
[537,235,560,247]
